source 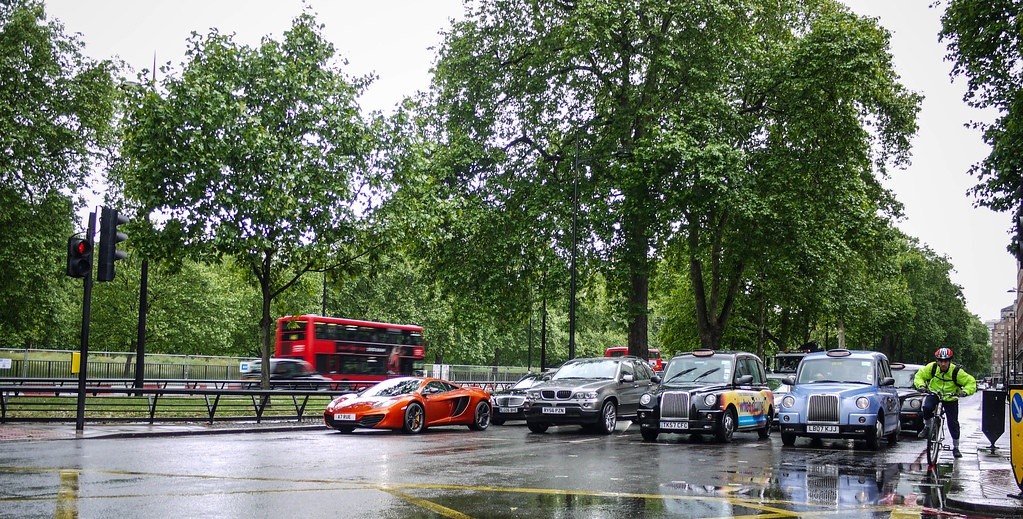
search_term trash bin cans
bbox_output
[982,389,1006,456]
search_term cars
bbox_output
[764,351,809,415]
[636,349,775,442]
[779,348,903,450]
[490,370,556,425]
[242,358,335,393]
[889,363,939,435]
[976,377,1005,390]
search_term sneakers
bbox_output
[953,447,962,457]
[918,426,930,438]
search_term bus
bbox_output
[606,348,663,373]
[274,314,424,391]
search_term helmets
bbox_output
[934,347,953,360]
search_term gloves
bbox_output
[918,386,928,393]
[957,390,966,397]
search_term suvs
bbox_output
[524,356,662,437]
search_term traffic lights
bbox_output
[67,236,92,279]
[97,209,129,283]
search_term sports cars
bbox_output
[326,378,495,434]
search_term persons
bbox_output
[913,348,976,457]
[387,347,401,375]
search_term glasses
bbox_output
[939,359,949,363]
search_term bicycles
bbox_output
[918,389,962,466]
